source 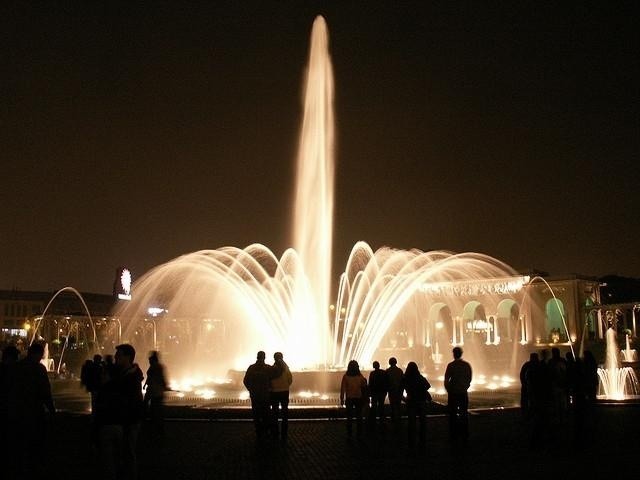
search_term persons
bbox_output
[368,362,386,433]
[404,362,430,434]
[444,347,471,441]
[520,346,600,455]
[0,333,173,480]
[386,358,404,424]
[270,354,293,448]
[244,350,282,448]
[341,360,367,440]
